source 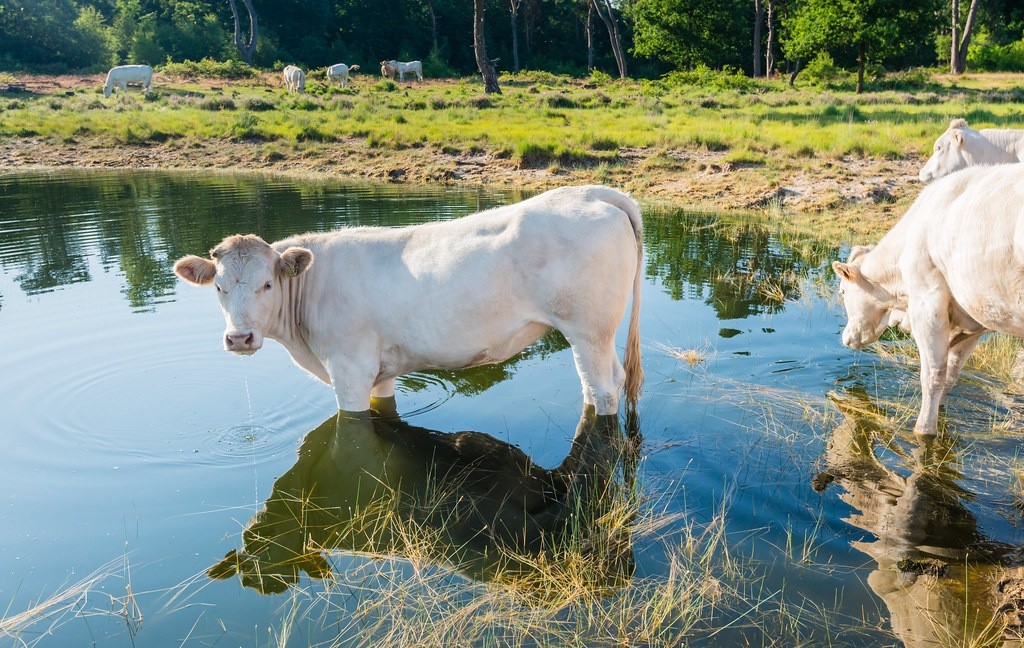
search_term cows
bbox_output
[327,63,360,88]
[103,65,153,99]
[283,65,308,94]
[204,405,644,597]
[380,59,425,83]
[917,119,1024,182]
[832,164,1023,438]
[174,185,647,415]
